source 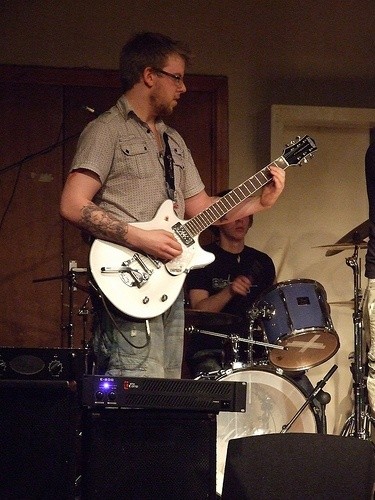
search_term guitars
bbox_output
[88,134,318,323]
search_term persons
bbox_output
[60,30,286,380]
[187,190,314,396]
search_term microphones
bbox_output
[79,104,102,117]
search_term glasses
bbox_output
[151,67,184,85]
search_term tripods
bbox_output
[340,258,375,441]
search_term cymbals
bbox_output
[312,218,371,257]
[329,298,356,308]
[185,309,244,326]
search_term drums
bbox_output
[193,360,322,497]
[254,279,339,371]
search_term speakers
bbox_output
[0,381,78,500]
[221,432,375,500]
[82,410,216,500]
